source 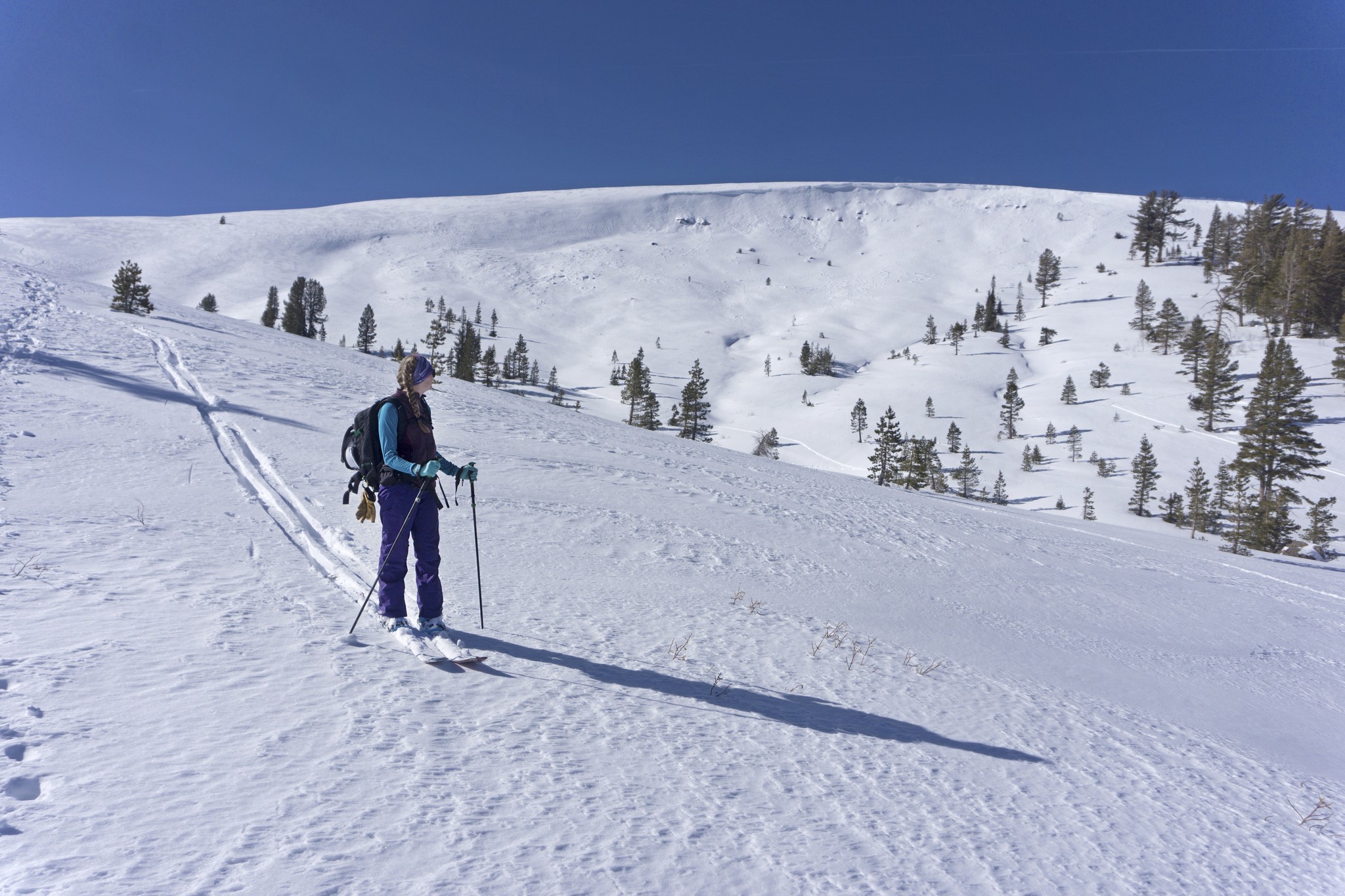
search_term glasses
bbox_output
[431,369,436,378]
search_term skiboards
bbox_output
[378,613,488,664]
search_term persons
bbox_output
[375,351,484,641]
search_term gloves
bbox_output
[412,460,440,477]
[454,465,478,481]
[364,489,376,523]
[355,491,367,523]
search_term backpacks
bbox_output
[340,394,434,505]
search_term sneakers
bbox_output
[380,617,411,633]
[419,618,448,638]
